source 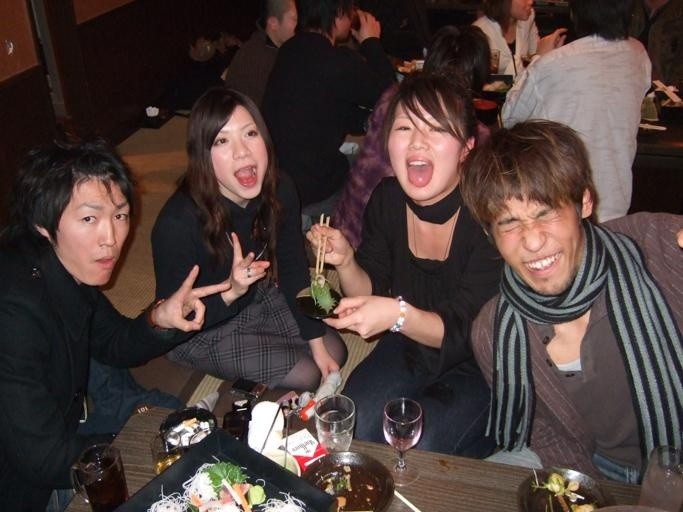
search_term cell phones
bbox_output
[231,377,267,401]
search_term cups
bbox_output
[635,447,682,512]
[148,431,182,477]
[69,444,129,512]
[490,47,501,74]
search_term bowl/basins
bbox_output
[257,450,300,476]
[299,452,394,512]
[159,407,216,446]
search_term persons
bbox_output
[220,0,682,486]
[1,119,237,511]
[149,83,352,395]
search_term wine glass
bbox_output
[313,393,356,455]
[379,395,422,489]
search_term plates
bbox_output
[515,466,617,512]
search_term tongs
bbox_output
[257,396,291,468]
